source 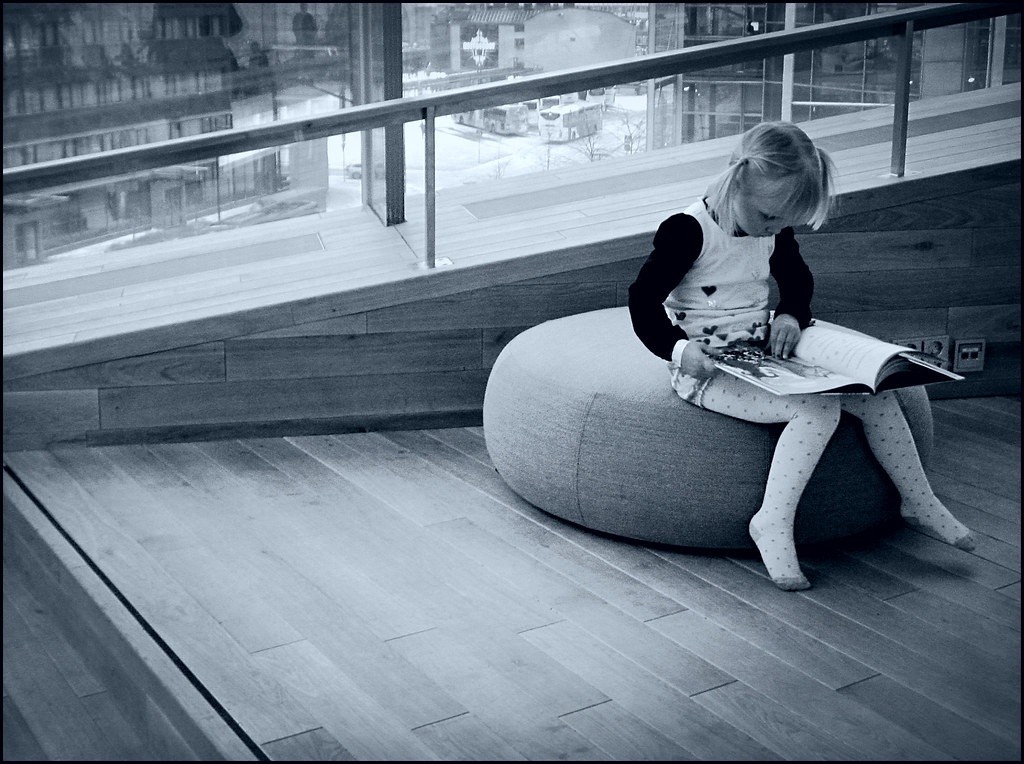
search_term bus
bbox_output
[538,101,603,142]
[585,85,616,111]
[518,92,579,126]
[451,104,528,136]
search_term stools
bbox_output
[485,307,935,551]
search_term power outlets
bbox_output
[893,336,950,372]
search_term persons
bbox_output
[626,121,977,591]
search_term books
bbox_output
[710,318,966,397]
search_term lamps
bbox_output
[966,73,975,83]
[747,22,759,36]
